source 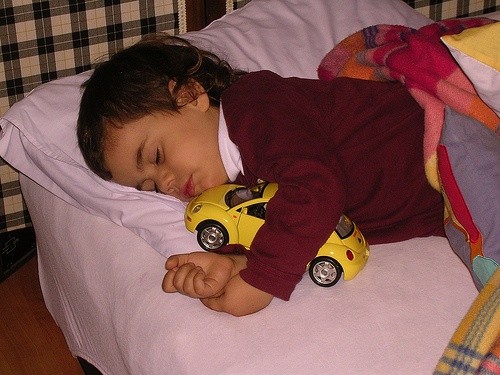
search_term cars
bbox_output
[185,181,371,288]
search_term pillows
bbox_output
[440,22,500,119]
[0,0,435,259]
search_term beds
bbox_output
[0,0,500,375]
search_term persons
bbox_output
[75,32,449,317]
